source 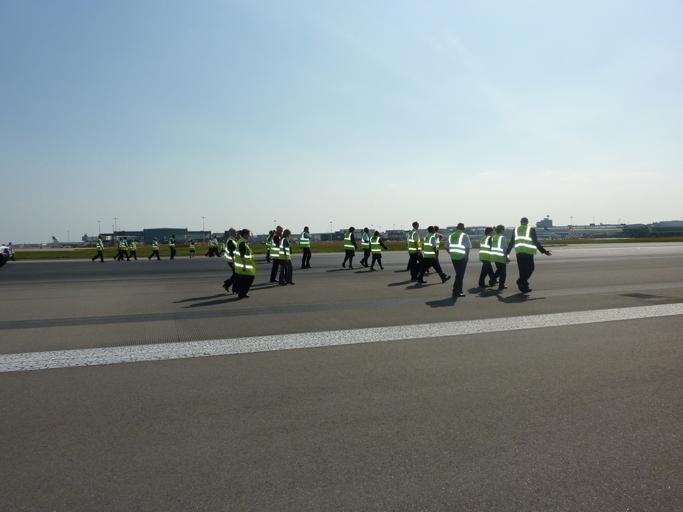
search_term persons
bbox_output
[265,226,295,286]
[342,226,358,270]
[506,218,551,293]
[370,230,388,270]
[0,242,15,266]
[91,234,227,263]
[360,227,372,268]
[299,226,312,269]
[479,224,511,290]
[445,223,473,298]
[406,221,451,284]
[223,227,256,298]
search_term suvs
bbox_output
[0,246,9,267]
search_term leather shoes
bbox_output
[443,276,451,282]
[418,280,427,283]
[454,293,465,297]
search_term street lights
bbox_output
[200,216,207,243]
[112,217,118,232]
[329,221,333,240]
[97,220,101,235]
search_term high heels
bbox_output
[223,283,229,292]
[232,288,236,295]
